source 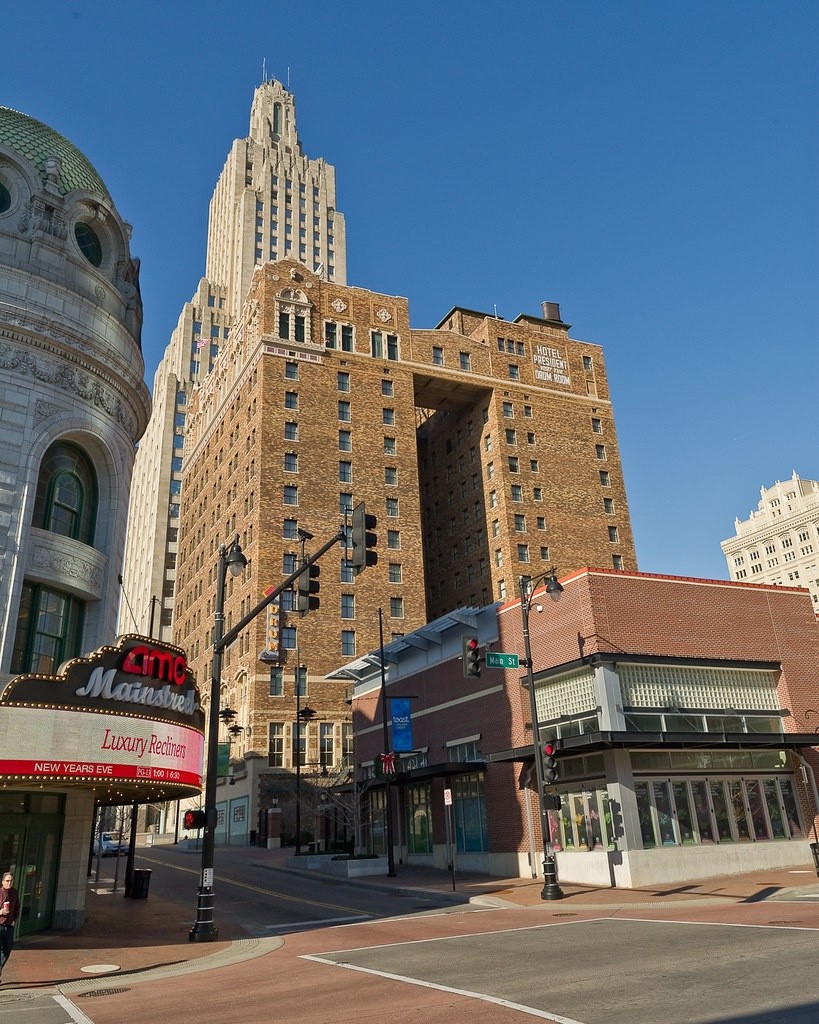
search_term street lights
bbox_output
[188,533,249,941]
[518,565,564,901]
[292,646,317,856]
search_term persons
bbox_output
[0,872,21,984]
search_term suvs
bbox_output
[93,832,131,857]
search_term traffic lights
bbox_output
[461,634,482,679]
[184,810,205,830]
[297,554,320,618]
[539,740,561,785]
[350,501,379,577]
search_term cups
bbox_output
[4,901,10,913]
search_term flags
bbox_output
[387,698,414,752]
[373,751,401,782]
[217,743,231,775]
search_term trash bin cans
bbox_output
[135,868,152,900]
